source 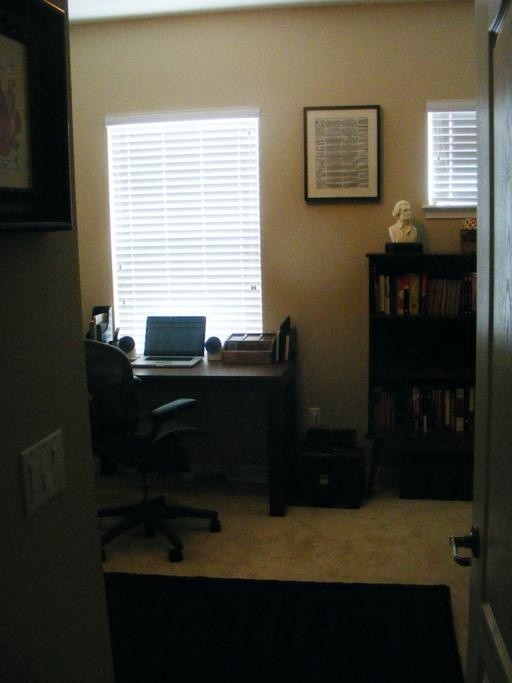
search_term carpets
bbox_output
[101,571,467,682]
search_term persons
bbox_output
[388,198,419,244]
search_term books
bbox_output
[374,266,477,313]
[372,382,476,431]
[90,312,108,341]
[272,315,297,362]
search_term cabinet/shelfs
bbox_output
[359,249,479,503]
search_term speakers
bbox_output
[206,337,223,361]
[119,336,136,361]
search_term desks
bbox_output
[123,354,305,521]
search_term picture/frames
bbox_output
[0,0,76,235]
[302,103,386,207]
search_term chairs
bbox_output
[80,335,225,565]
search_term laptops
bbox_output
[130,317,206,368]
